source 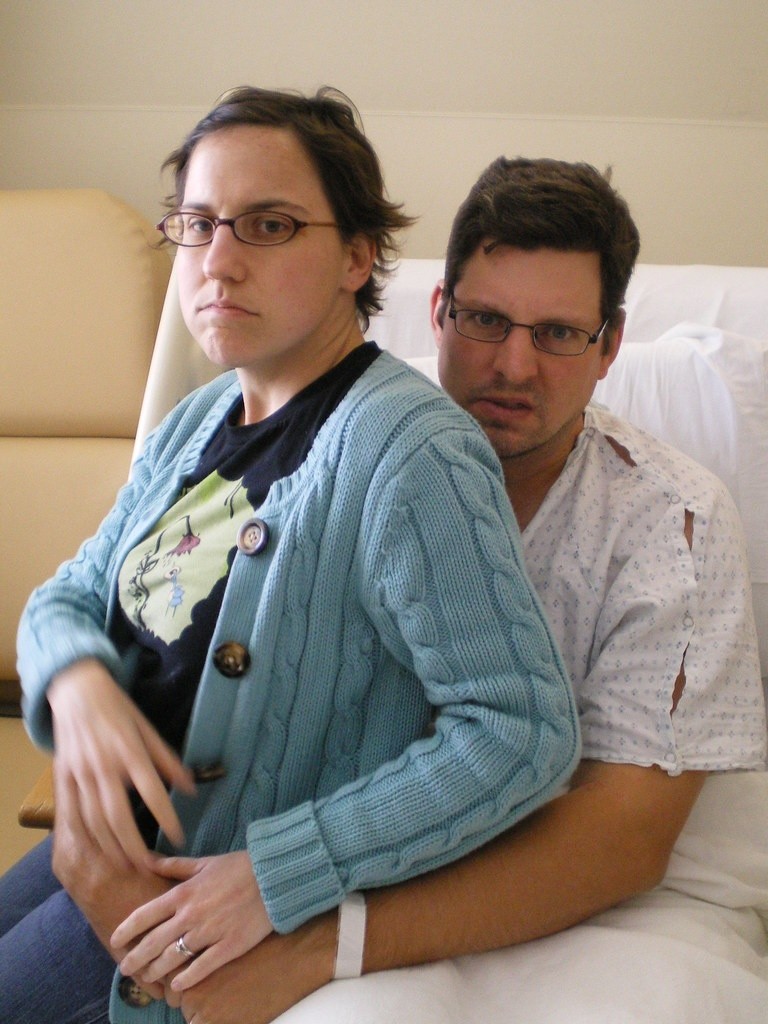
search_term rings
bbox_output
[176,936,195,959]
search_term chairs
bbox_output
[1,190,161,873]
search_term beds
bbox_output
[128,237,767,1022]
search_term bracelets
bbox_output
[333,892,367,980]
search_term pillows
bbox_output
[401,329,768,588]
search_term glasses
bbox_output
[154,211,340,247]
[448,289,610,357]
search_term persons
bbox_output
[51,156,767,1024]
[0,85,583,1024]
